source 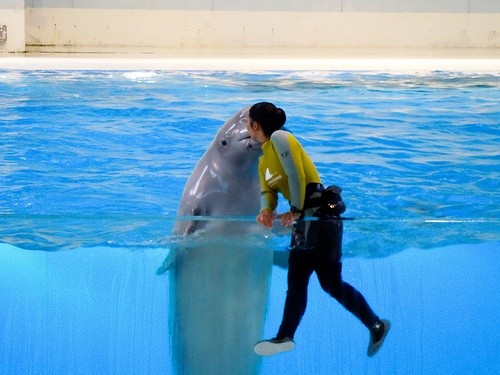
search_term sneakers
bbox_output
[366,318,392,358]
[252,336,297,357]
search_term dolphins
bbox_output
[154,104,273,375]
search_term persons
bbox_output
[246,102,391,358]
